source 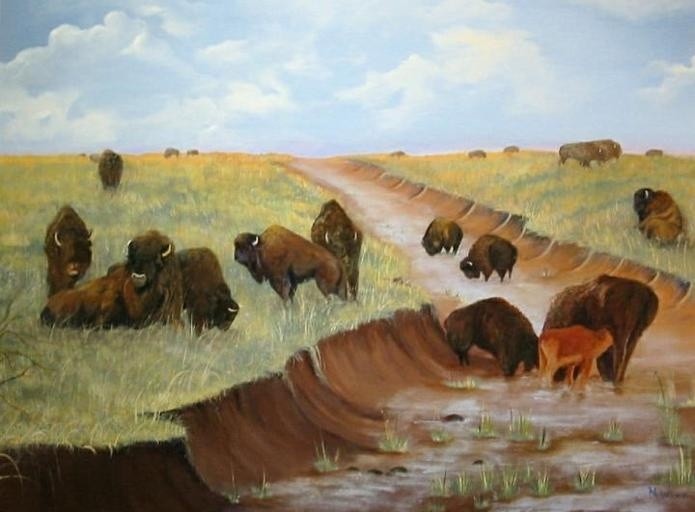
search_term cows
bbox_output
[39,204,239,338]
[633,188,683,242]
[98,149,123,200]
[421,216,463,255]
[559,140,622,167]
[459,234,518,282]
[443,274,658,391]
[233,224,344,306]
[310,199,363,301]
